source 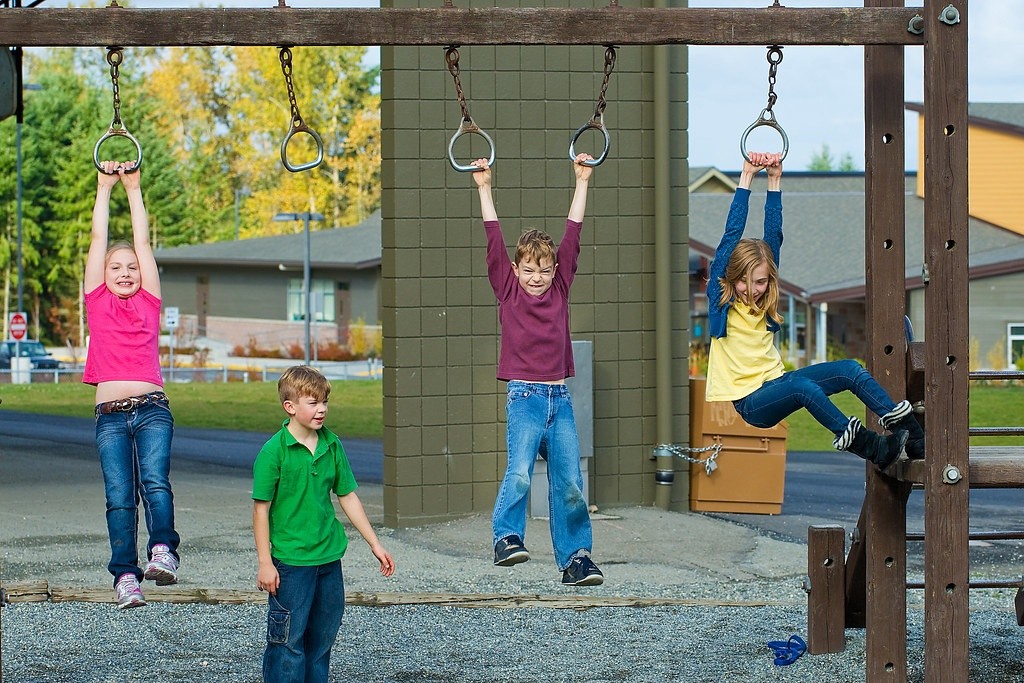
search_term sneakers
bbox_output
[114,572,146,608]
[144,544,180,586]
[494,536,530,566]
[562,556,603,586]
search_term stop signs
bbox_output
[9,311,27,342]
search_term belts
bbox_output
[95,393,166,414]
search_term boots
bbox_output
[878,400,925,458]
[832,415,908,471]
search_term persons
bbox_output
[471,153,605,586]
[82,160,180,609]
[705,151,924,470]
[252,365,394,683]
